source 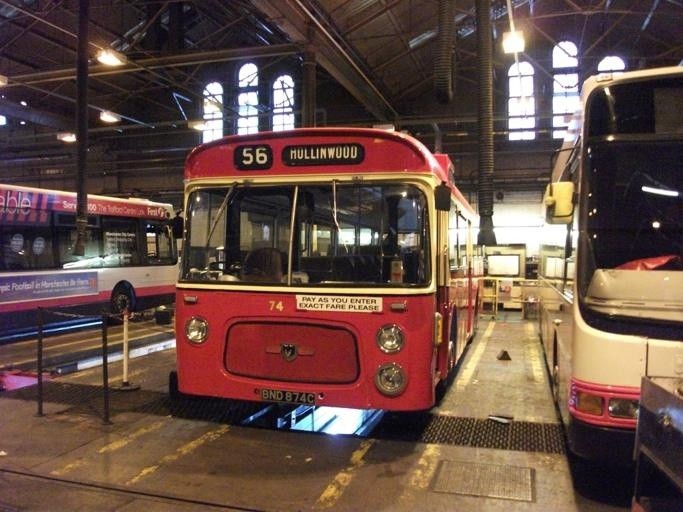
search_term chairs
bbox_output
[299,243,416,286]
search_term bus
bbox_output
[0,183,179,325]
[540,66,683,459]
[172,123,485,410]
